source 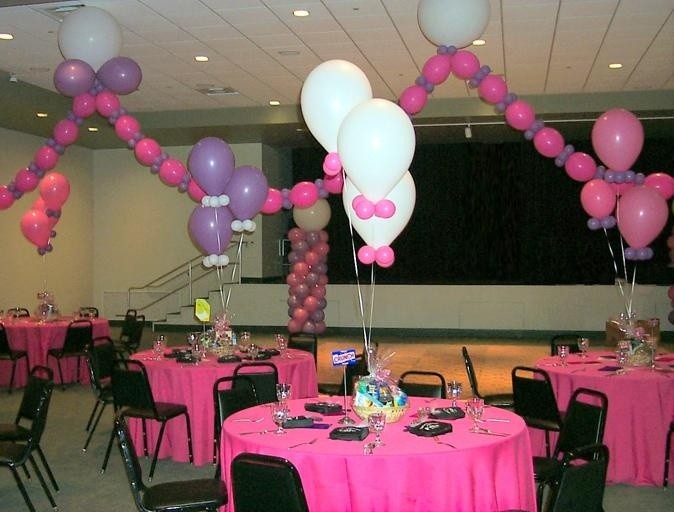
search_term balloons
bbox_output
[57,7,123,73]
[2,46,674,337]
[417,0,490,49]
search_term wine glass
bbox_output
[559,337,673,376]
[231,382,512,453]
[153,332,291,366]
[0,306,96,324]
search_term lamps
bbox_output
[465,124,472,138]
